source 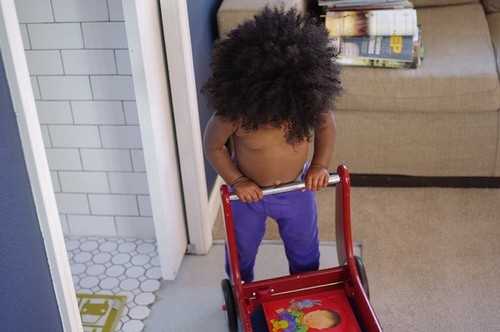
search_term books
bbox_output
[316,0,425,71]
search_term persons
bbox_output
[200,5,337,286]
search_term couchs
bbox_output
[217,0,500,189]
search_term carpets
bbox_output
[142,241,363,332]
